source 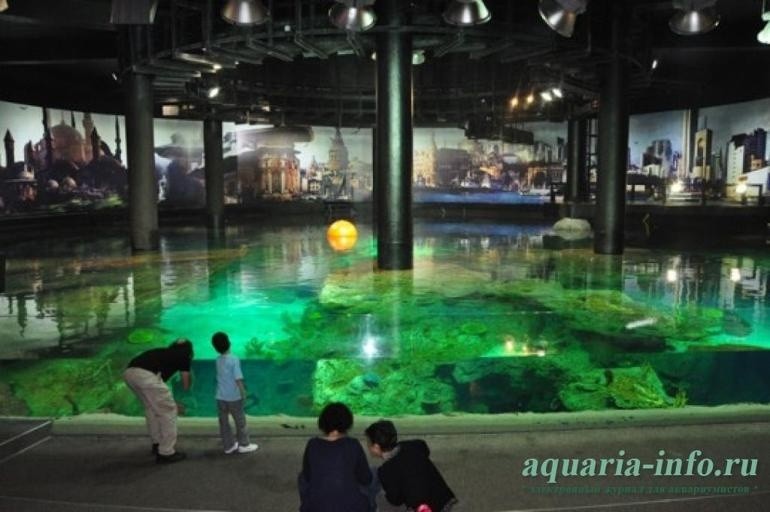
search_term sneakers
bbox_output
[224,441,259,454]
[152,443,186,465]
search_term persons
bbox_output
[298,402,378,510]
[209,331,259,454]
[123,335,195,465]
[363,419,458,511]
